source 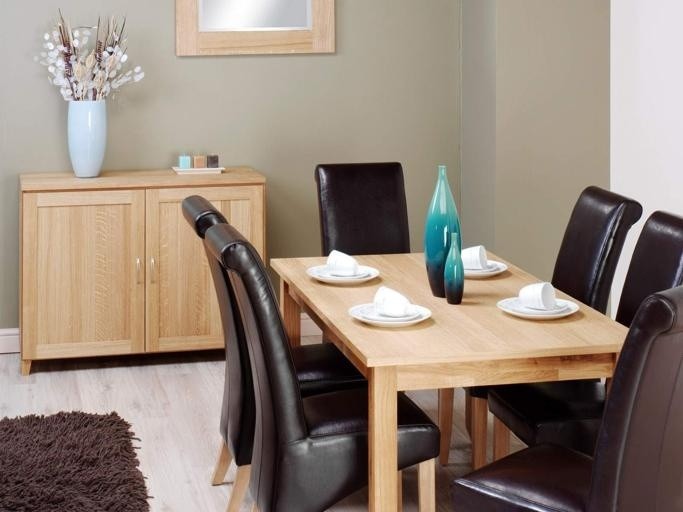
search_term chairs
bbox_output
[451,285,683,511]
[181,195,370,512]
[462,184,644,468]
[205,226,442,512]
[487,211,683,463]
[314,161,413,342]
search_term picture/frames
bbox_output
[173,0,337,58]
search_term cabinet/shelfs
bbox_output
[20,166,267,376]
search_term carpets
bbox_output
[2,409,154,511]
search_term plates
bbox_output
[464,260,508,279]
[306,264,380,285]
[496,297,580,320]
[348,303,432,328]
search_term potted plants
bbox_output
[33,7,144,178]
